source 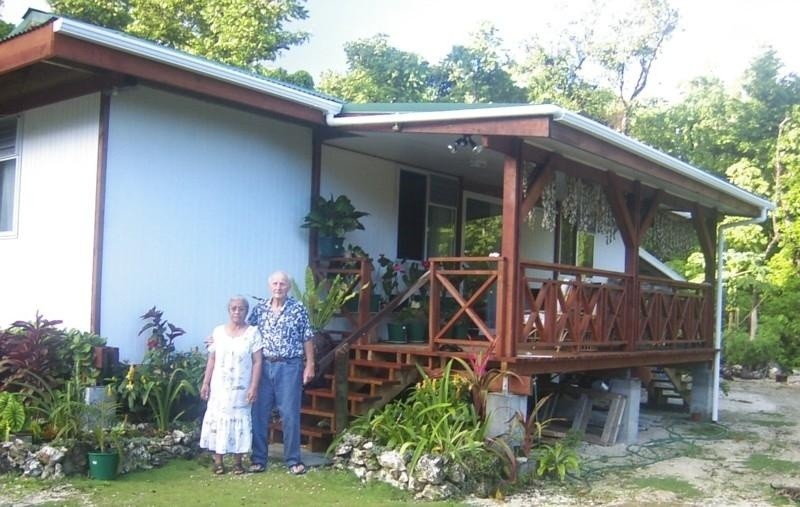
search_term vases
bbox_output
[388,316,427,343]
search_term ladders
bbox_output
[532,381,626,446]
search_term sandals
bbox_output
[233,463,245,474]
[214,463,225,474]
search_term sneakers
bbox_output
[289,463,305,474]
[248,463,264,473]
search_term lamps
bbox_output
[446,134,484,153]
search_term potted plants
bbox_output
[77,400,127,481]
[293,267,358,360]
[440,261,488,340]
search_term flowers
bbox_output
[376,254,430,321]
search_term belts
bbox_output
[265,357,286,362]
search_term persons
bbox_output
[205,270,316,475]
[199,293,265,477]
